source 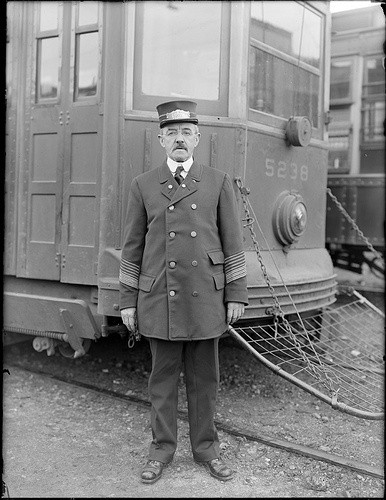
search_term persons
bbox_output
[116,100,251,485]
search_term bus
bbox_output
[3,0,386,423]
[323,4,385,283]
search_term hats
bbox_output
[156,100,201,127]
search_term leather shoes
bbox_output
[139,459,166,484]
[196,455,234,481]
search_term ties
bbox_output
[174,166,187,186]
